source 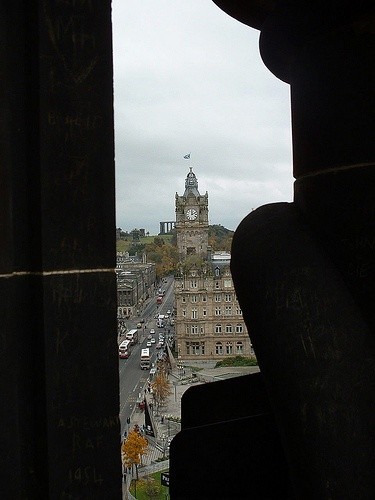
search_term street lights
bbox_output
[127,417,130,436]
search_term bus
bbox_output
[119,339,131,360]
[140,348,150,369]
[126,329,138,345]
[157,297,162,305]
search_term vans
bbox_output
[155,318,164,328]
[159,314,164,319]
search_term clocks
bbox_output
[186,208,198,220]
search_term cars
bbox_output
[147,334,152,340]
[159,333,164,339]
[151,339,155,343]
[164,314,169,318]
[117,321,128,336]
[158,290,166,297]
[158,339,163,345]
[149,329,155,334]
[140,318,144,323]
[147,341,151,346]
[166,310,171,314]
[153,313,159,318]
[180,378,199,385]
[137,323,141,329]
[155,344,161,349]
